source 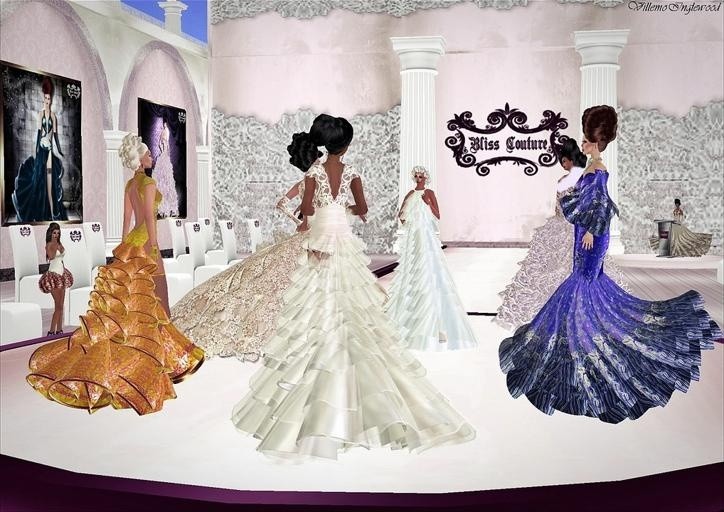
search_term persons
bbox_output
[27,113,478,459]
[499,105,723,425]
[12,75,64,221]
